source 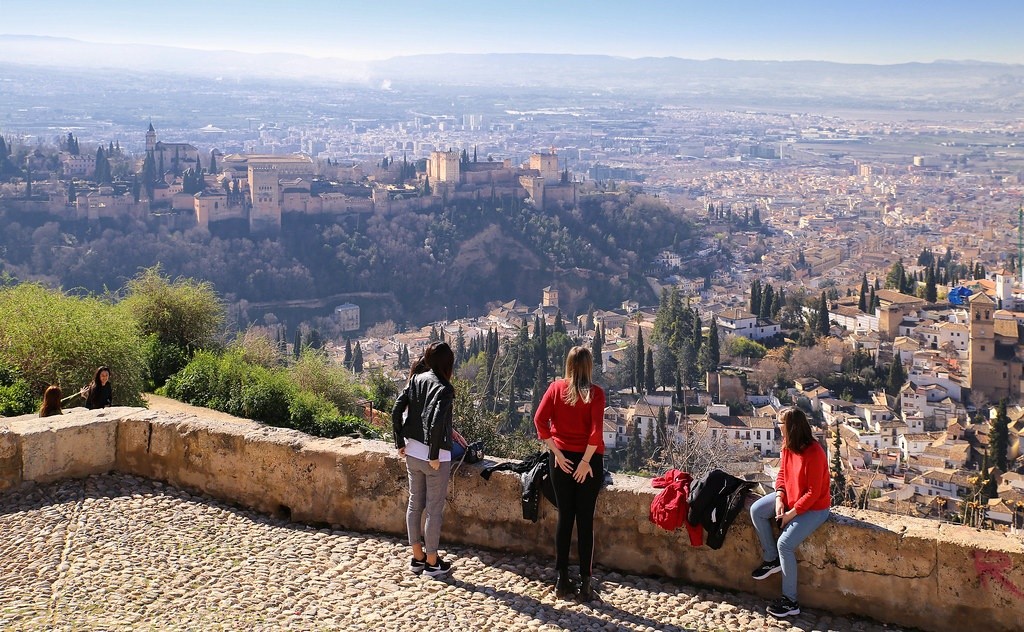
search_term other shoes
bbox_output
[555,578,577,596]
[577,584,594,602]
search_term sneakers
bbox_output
[751,556,782,580]
[423,556,451,576]
[409,552,427,572]
[766,595,800,617]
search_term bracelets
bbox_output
[581,459,590,463]
[776,490,786,495]
[775,495,781,497]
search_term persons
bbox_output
[392,341,455,577]
[80,366,112,411]
[749,405,831,618]
[39,385,90,418]
[533,346,605,602]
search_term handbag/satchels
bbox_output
[450,440,465,461]
[463,438,485,465]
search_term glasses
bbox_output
[775,422,785,428]
